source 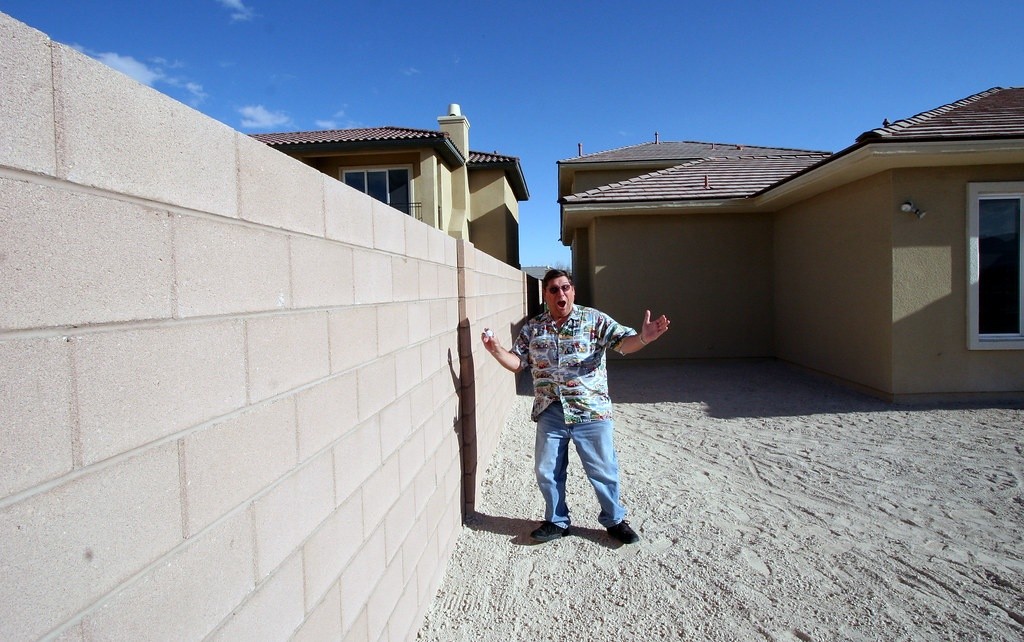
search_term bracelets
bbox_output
[639,333,647,346]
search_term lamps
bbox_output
[900,200,928,218]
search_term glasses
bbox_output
[545,285,571,294]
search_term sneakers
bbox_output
[530,521,570,541]
[607,520,639,543]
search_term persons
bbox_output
[482,269,670,544]
